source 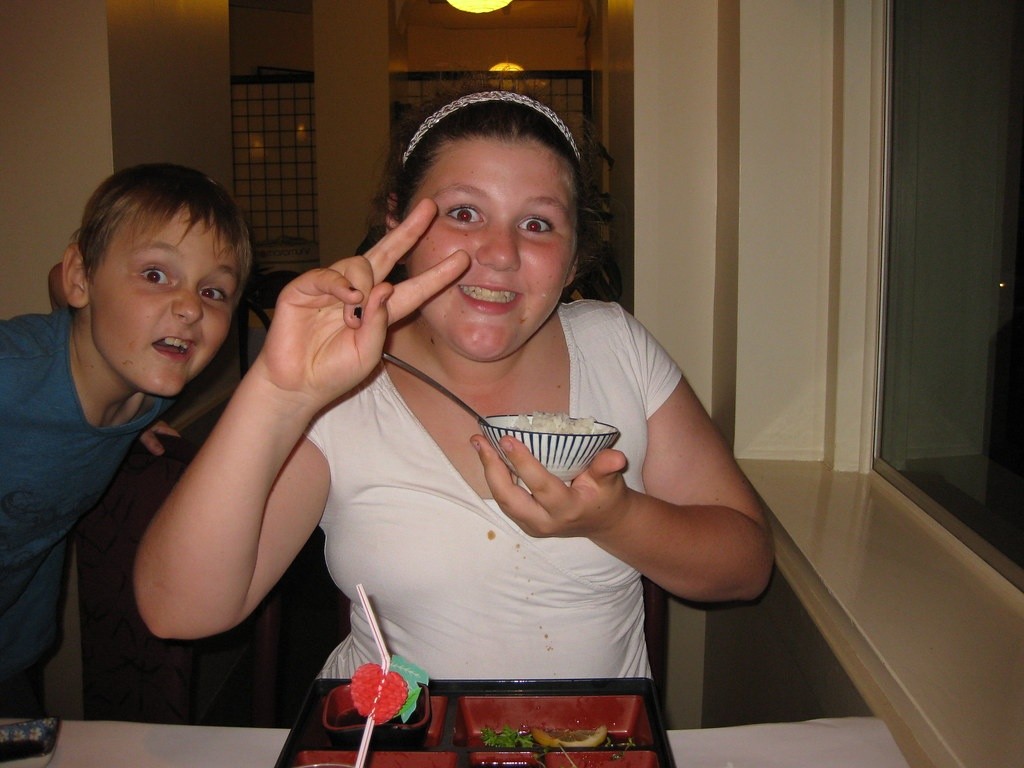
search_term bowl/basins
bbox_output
[479,412,620,499]
[323,682,434,751]
[0,715,60,768]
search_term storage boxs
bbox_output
[273,677,679,768]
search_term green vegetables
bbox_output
[480,724,637,768]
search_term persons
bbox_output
[0,161,253,758]
[133,85,775,675]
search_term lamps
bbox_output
[488,62,524,72]
[446,0,513,14]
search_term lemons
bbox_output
[530,725,607,747]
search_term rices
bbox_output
[506,410,609,433]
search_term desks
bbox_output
[0,716,911,768]
